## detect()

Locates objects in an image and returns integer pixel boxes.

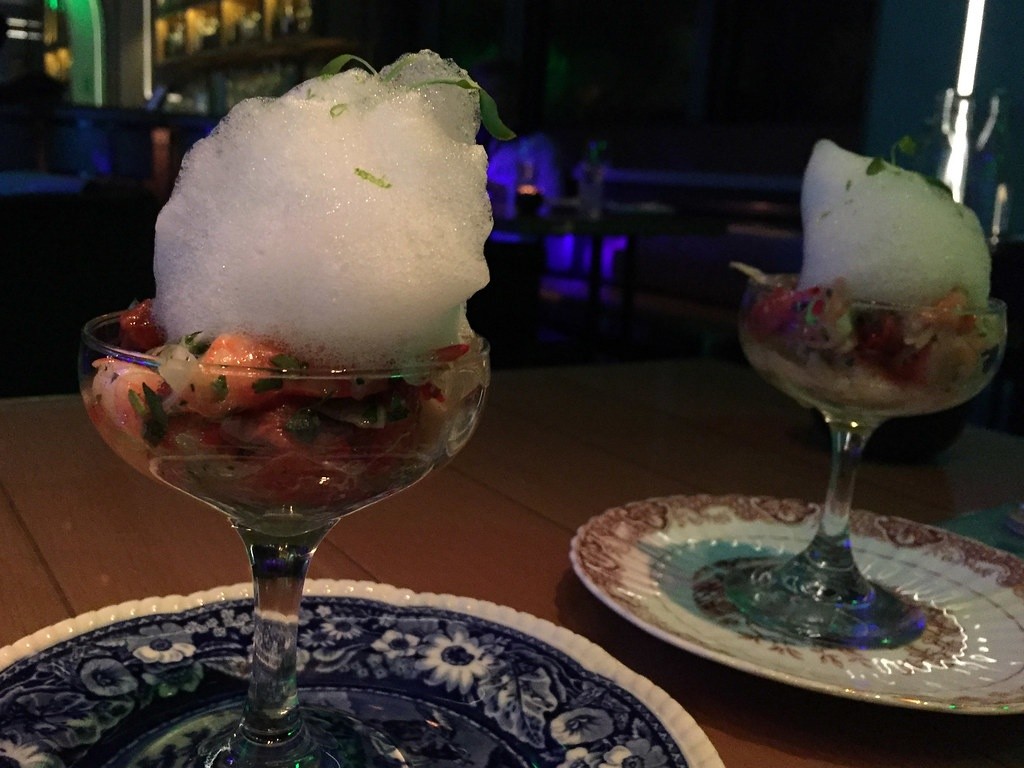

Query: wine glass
[720,283,1009,650]
[75,303,492,768]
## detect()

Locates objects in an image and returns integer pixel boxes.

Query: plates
[1,576,724,768]
[567,493,1024,717]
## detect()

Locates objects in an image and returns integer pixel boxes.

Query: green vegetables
[126,52,515,444]
[865,138,955,203]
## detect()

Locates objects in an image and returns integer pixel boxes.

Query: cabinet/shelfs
[151,1,355,122]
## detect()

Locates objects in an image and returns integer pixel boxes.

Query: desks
[494,207,724,361]
[0,165,136,211]
[1,364,1024,768]
